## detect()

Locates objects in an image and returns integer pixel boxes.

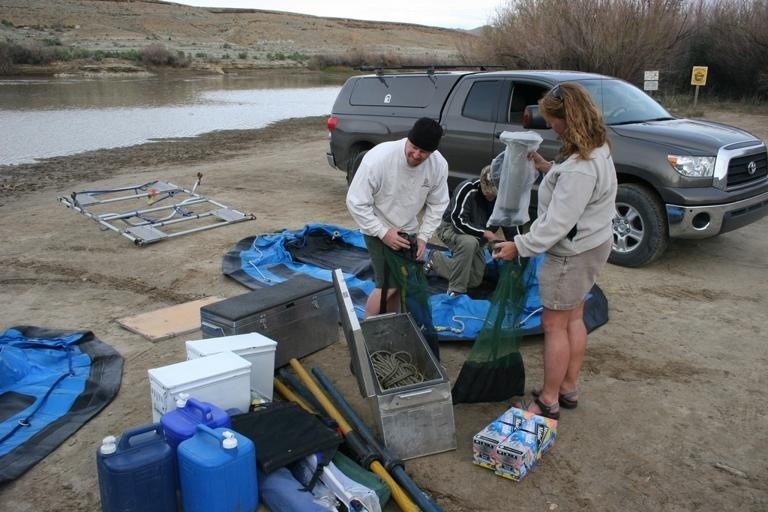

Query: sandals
[532,382,578,410]
[509,397,560,421]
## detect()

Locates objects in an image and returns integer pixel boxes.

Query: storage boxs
[147,350,252,424]
[186,333,278,405]
[332,269,457,461]
[200,273,339,369]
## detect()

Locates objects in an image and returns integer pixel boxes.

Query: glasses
[552,83,564,105]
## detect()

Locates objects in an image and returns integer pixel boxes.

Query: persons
[345,117,452,320]
[487,81,620,422]
[423,164,525,294]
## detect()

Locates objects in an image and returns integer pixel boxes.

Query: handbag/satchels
[231,402,340,471]
[258,448,391,512]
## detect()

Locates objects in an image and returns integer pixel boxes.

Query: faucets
[177,393,191,408]
[220,429,238,449]
[98,434,116,454]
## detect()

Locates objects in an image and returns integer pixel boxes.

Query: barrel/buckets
[176,422,259,512]
[159,395,230,446]
[95,420,175,512]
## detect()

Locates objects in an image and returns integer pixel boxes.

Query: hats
[408,117,443,152]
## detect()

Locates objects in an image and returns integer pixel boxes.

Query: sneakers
[447,287,461,297]
[423,249,439,275]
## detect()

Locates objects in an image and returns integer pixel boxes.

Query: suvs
[325,65,766,268]
